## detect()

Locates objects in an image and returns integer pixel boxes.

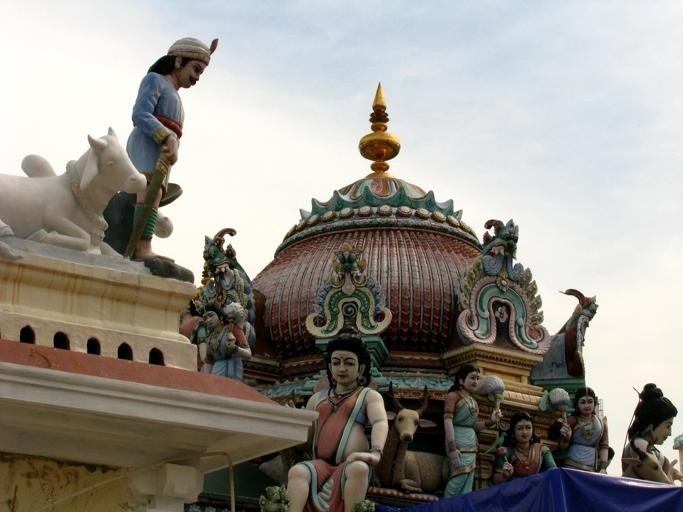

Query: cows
[373,380,438,493]
[1,125,148,260]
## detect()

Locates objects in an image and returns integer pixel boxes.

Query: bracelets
[230,344,239,355]
[369,447,384,458]
[561,434,569,445]
[448,441,456,452]
[197,336,208,345]
[485,420,495,427]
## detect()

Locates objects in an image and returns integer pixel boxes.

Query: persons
[620,383,682,483]
[557,387,609,475]
[490,410,559,483]
[442,363,504,499]
[285,323,390,512]
[194,302,252,383]
[125,35,220,263]
